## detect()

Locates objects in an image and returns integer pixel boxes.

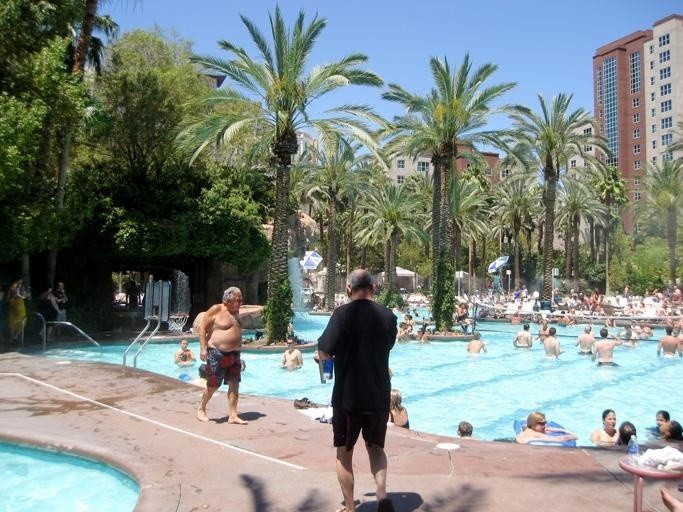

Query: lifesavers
[514,416,576,447]
[645,425,663,441]
[176,358,196,367]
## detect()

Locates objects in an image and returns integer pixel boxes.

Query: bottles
[626,434,641,469]
[323,358,334,380]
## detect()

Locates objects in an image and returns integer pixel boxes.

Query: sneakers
[294,398,319,409]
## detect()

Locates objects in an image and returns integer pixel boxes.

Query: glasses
[537,421,546,425]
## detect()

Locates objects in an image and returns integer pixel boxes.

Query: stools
[618,454,683,512]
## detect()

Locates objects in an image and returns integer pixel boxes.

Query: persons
[172,279,683,449]
[124,281,144,329]
[53,282,69,336]
[658,485,683,512]
[6,274,33,346]
[37,285,63,341]
[312,268,400,511]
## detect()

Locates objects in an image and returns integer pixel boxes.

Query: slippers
[377,498,395,512]
[337,509,357,512]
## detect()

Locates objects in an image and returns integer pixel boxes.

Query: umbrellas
[299,250,324,271]
[488,256,510,274]
